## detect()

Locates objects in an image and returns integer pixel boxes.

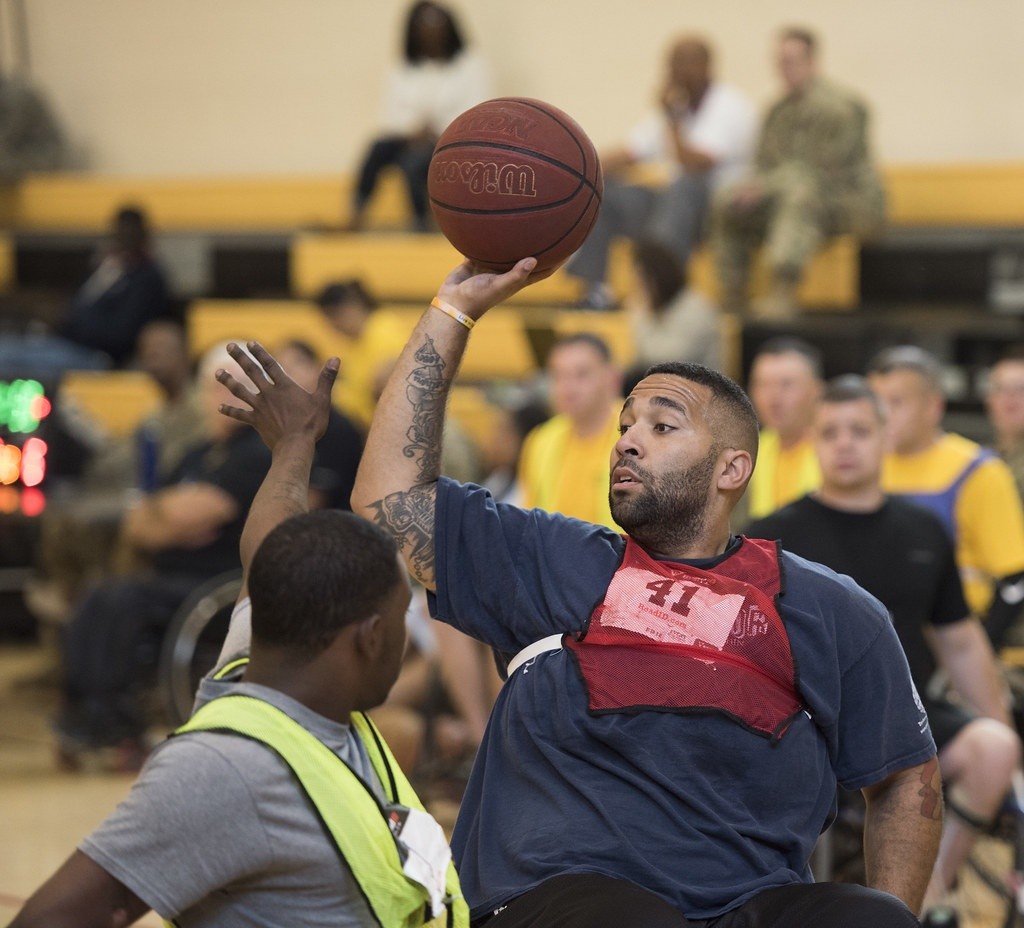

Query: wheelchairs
[29,541,1024,928]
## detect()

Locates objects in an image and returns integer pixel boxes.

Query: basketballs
[426,94,604,277]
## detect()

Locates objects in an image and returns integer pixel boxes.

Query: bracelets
[433,298,475,327]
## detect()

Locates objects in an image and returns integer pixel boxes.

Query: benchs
[0,169,1021,905]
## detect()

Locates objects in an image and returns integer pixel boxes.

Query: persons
[747,374,1018,928]
[7,340,470,928]
[349,1,495,229]
[565,32,884,323]
[351,257,943,928]
[0,208,1024,928]
